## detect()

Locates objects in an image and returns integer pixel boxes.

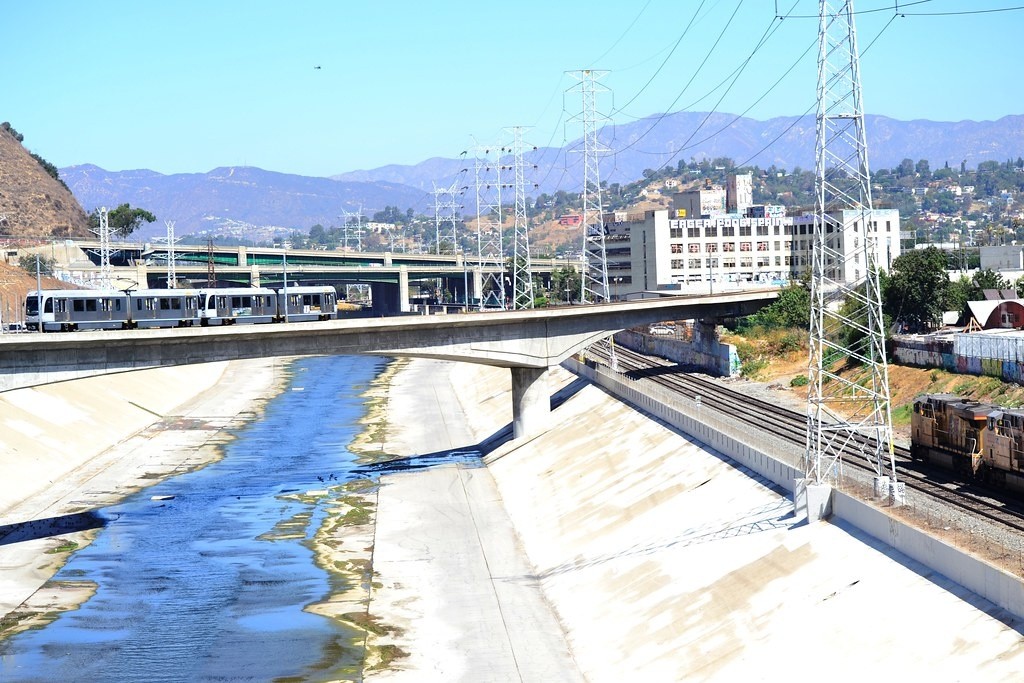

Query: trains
[910,393,1024,499]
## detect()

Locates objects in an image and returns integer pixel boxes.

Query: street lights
[614,275,623,302]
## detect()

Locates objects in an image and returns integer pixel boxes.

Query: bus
[22,285,337,333]
